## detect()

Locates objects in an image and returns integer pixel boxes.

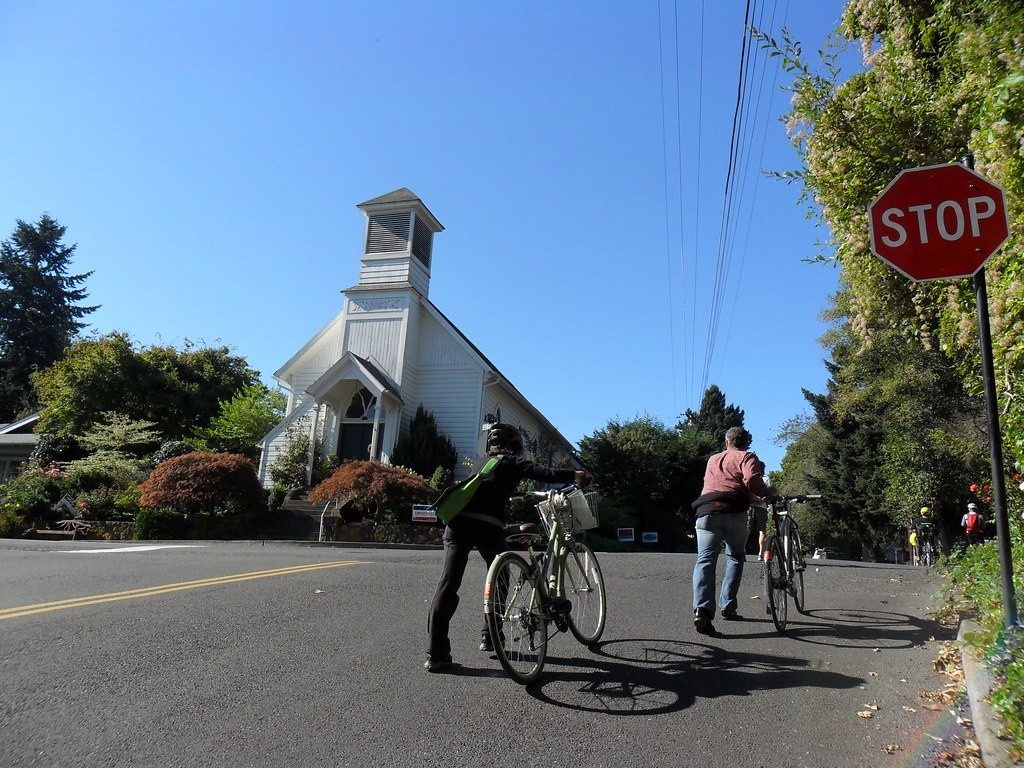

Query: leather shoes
[693,607,714,633]
[721,605,738,619]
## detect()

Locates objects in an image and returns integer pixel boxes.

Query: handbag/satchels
[428,472,484,525]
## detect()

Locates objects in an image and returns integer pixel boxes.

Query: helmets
[485,423,524,454]
[920,506,931,515]
[967,503,977,509]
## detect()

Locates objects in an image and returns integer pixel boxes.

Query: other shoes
[424,649,454,671]
[915,556,920,560]
[480,634,506,652]
[758,554,763,561]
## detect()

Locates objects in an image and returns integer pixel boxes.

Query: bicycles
[482,475,610,686]
[909,518,934,566]
[749,491,828,632]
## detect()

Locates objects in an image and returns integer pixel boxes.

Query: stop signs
[864,160,1011,289]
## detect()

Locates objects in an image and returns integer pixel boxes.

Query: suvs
[811,547,823,560]
[820,546,841,559]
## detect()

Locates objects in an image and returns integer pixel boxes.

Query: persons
[961,503,985,547]
[425,421,584,670]
[743,461,783,561]
[909,507,941,562]
[692,426,779,633]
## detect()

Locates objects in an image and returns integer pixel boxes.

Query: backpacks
[966,512,979,532]
